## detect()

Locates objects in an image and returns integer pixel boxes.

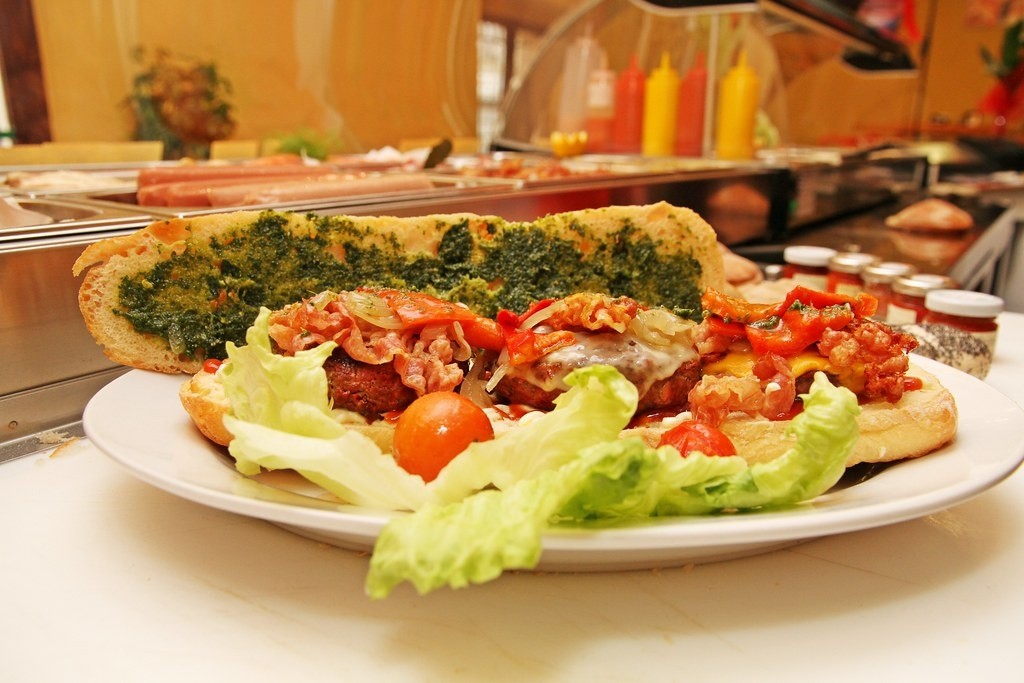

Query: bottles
[560,22,760,158]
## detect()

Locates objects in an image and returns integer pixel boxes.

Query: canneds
[783,244,1004,383]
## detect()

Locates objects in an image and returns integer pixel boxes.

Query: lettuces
[210,304,864,604]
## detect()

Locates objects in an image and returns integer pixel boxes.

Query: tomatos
[391,389,495,484]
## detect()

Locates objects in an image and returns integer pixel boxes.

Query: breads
[181,361,958,472]
[69,200,724,376]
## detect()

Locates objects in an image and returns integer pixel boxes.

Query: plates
[81,351,1024,572]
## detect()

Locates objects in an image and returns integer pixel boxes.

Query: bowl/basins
[0,155,724,241]
